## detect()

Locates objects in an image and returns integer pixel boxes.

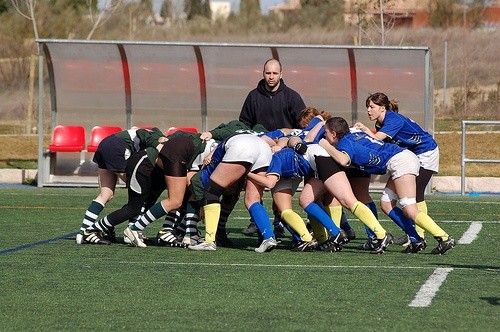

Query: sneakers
[392,236,410,245]
[123,227,146,248]
[357,237,373,250]
[181,234,204,245]
[432,236,456,255]
[172,230,185,242]
[288,238,319,251]
[273,224,285,237]
[107,229,117,243]
[313,238,342,252]
[215,229,232,247]
[188,240,216,251]
[241,222,258,236]
[254,237,278,253]
[156,229,182,246]
[371,232,394,254]
[83,229,111,245]
[346,228,356,241]
[404,239,426,253]
[328,230,350,248]
[75,234,84,245]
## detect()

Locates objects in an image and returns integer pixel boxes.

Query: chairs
[58,57,427,109]
[48,126,197,174]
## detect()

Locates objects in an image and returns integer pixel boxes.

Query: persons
[320,117,456,254]
[240,59,306,237]
[77,107,407,252]
[355,92,439,246]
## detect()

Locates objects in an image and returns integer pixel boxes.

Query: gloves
[295,143,308,155]
